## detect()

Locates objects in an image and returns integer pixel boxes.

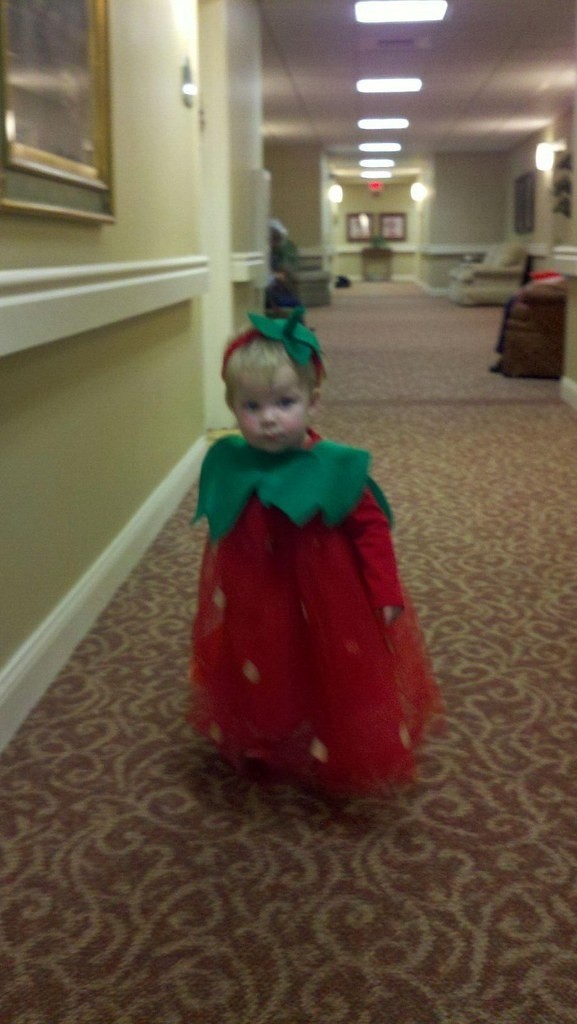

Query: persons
[189,305,443,796]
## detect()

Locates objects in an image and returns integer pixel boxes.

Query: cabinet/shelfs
[505,289,564,382]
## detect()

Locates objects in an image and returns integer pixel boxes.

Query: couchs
[449,244,528,306]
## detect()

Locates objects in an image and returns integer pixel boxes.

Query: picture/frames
[378,212,407,242]
[0,0,118,226]
[346,212,374,242]
